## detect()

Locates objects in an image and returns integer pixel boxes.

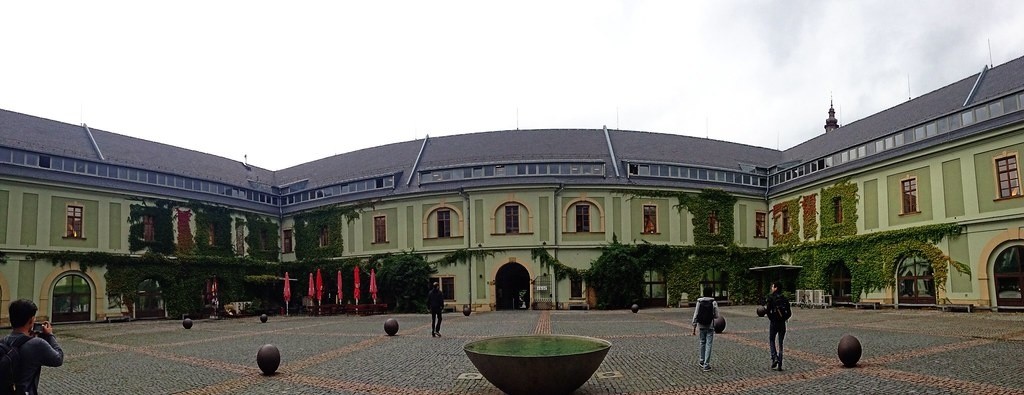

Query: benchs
[854,302,879,310]
[679,301,697,307]
[717,301,731,306]
[108,316,130,323]
[937,304,973,313]
[568,304,589,310]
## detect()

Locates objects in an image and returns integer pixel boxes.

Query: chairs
[306,304,388,316]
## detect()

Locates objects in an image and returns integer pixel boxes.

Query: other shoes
[778,365,783,371]
[432,332,435,337]
[436,332,441,336]
[771,357,780,368]
[703,364,712,372]
[699,362,704,367]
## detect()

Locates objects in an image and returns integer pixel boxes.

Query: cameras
[31,322,47,333]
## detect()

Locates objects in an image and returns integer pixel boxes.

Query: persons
[766,282,791,371]
[692,286,720,371]
[0,298,63,395]
[66,295,71,312]
[426,282,444,338]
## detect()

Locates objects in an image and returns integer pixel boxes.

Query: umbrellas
[308,273,316,313]
[369,269,378,305]
[338,270,343,306]
[283,272,291,315]
[353,266,360,314]
[315,269,323,315]
[211,278,218,316]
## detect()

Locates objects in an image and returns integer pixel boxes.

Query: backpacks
[769,294,791,322]
[697,300,715,325]
[0,335,38,395]
